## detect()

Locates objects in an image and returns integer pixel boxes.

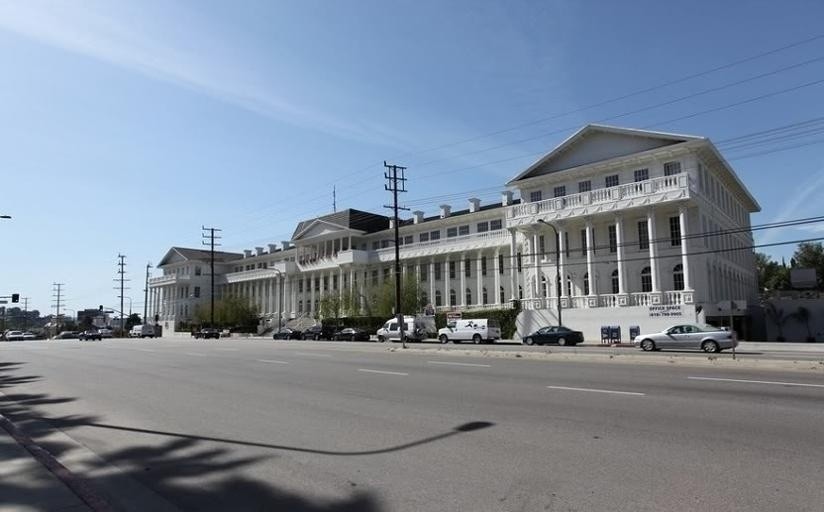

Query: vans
[376,315,429,343]
[438,318,502,347]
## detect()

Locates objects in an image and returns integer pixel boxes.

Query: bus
[321,315,391,341]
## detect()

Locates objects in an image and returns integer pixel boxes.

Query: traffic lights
[99,305,103,312]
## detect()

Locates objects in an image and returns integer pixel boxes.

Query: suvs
[128,325,155,339]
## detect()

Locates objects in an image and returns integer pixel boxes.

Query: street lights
[117,295,132,320]
[63,308,75,320]
[262,267,281,333]
[536,218,562,326]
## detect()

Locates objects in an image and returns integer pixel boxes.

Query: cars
[191,328,220,339]
[521,325,583,348]
[0,327,115,341]
[633,321,738,354]
[301,324,324,340]
[273,327,301,340]
[333,327,370,341]
